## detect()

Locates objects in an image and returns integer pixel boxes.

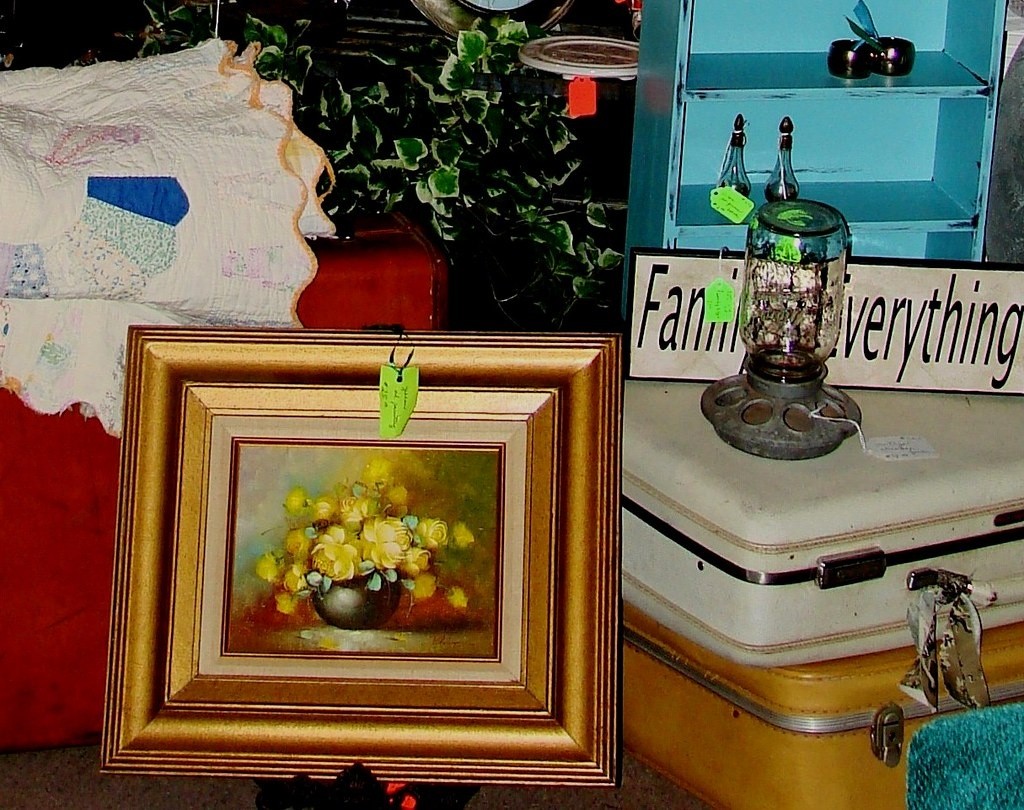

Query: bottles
[716,114,751,200]
[763,117,800,203]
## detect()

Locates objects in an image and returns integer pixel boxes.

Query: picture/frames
[101,320,624,789]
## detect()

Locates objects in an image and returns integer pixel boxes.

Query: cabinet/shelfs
[622,0,1007,326]
